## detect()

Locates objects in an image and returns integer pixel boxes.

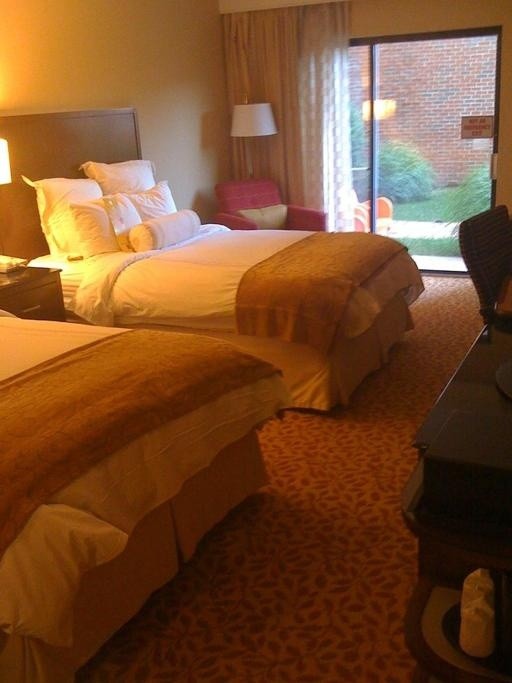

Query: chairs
[458,204,511,331]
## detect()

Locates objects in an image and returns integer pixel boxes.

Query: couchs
[352,188,393,232]
[213,178,327,229]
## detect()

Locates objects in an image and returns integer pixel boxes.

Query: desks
[397,318,510,682]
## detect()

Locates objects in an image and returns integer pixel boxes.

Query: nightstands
[1,266,67,322]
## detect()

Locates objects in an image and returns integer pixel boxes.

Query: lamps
[230,102,279,177]
[0,136,14,184]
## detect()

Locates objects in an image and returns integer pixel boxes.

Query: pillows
[129,207,202,254]
[91,191,145,252]
[77,158,156,195]
[66,196,120,258]
[235,202,288,230]
[128,179,178,221]
[21,175,102,259]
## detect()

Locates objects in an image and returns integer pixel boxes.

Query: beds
[0,309,285,683]
[27,223,425,413]
